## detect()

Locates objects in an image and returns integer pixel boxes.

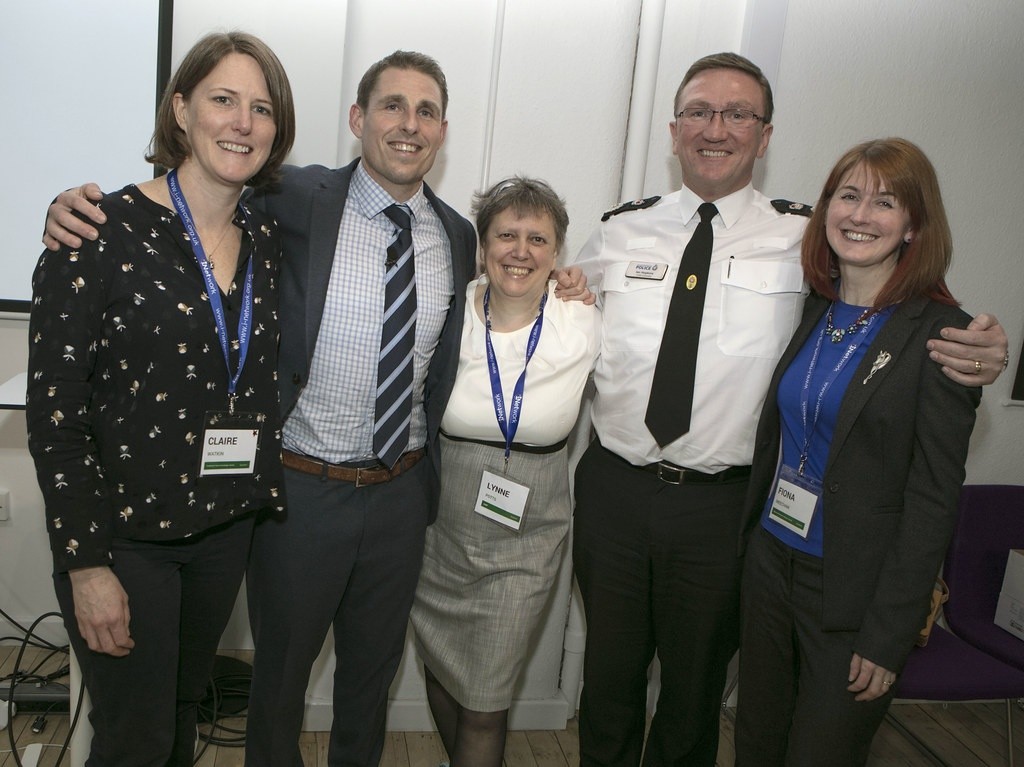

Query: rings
[975,361,981,375]
[884,682,892,685]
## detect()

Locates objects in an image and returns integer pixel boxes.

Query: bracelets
[1003,351,1008,371]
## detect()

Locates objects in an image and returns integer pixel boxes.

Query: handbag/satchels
[916,576,949,646]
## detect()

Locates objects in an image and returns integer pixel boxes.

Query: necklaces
[196,216,233,270]
[826,301,881,343]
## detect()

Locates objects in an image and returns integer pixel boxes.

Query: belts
[603,448,751,486]
[282,448,426,488]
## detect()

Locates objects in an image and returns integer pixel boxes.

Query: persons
[407,175,603,767]
[25,31,285,767]
[569,53,1007,767]
[41,51,594,767]
[734,135,983,767]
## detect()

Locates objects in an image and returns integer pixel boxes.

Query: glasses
[675,108,766,128]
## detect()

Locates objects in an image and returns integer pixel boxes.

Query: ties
[372,204,417,470]
[644,202,720,448]
